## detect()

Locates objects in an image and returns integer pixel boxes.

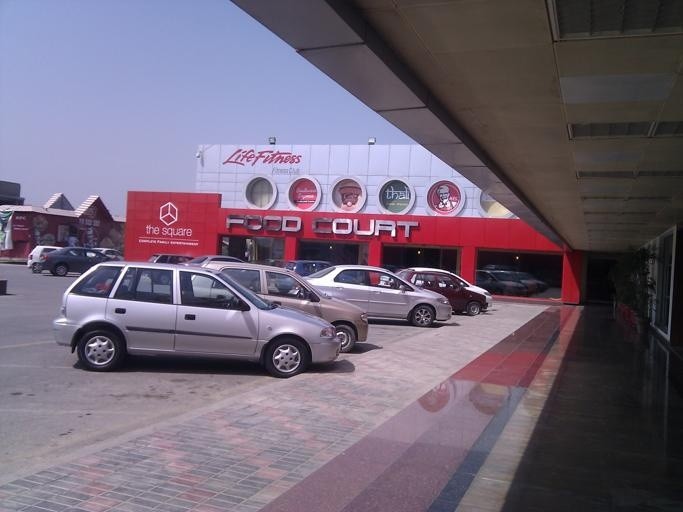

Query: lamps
[368,136,376,144]
[268,136,277,144]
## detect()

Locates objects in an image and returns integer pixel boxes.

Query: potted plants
[615,246,658,335]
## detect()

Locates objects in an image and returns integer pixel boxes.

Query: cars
[27,246,124,277]
[475,267,549,296]
